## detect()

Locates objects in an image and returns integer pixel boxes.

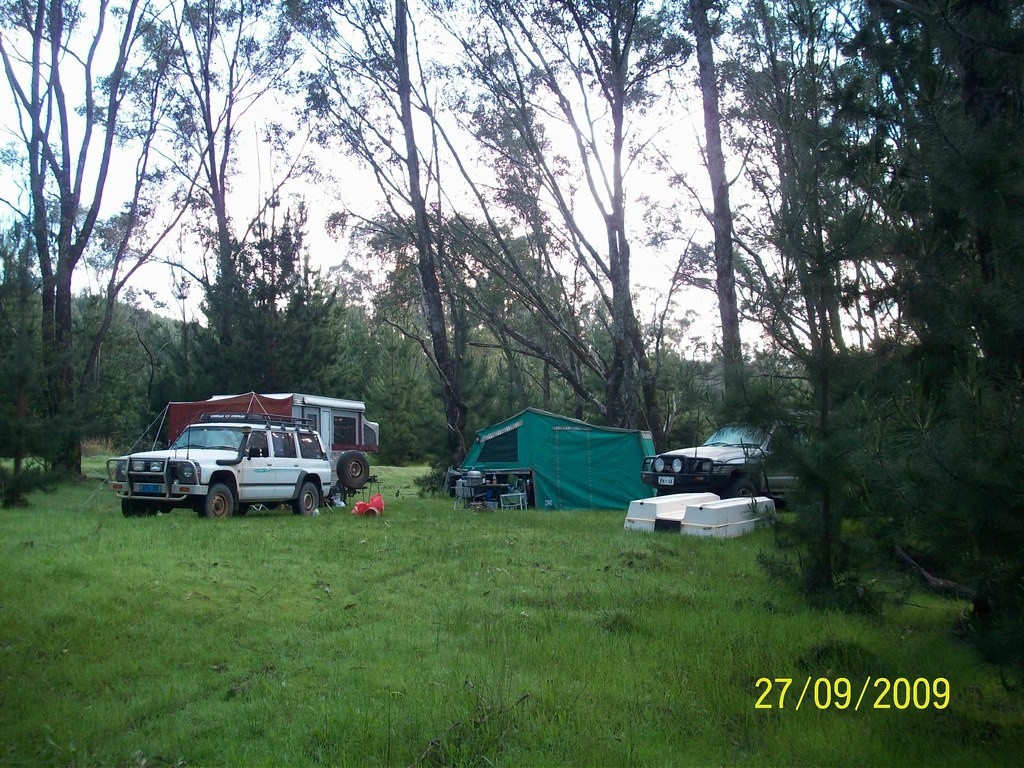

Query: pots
[467,471,482,485]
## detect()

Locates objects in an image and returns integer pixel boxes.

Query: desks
[451,483,529,510]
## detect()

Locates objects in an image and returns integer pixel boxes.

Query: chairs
[454,479,486,510]
[501,478,527,512]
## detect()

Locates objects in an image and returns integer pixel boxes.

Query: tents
[458,407,658,510]
[166,391,294,447]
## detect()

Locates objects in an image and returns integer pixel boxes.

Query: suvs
[640,410,839,509]
[106,411,331,520]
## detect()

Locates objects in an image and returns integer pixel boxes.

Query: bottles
[482,475,486,485]
[493,474,497,485]
